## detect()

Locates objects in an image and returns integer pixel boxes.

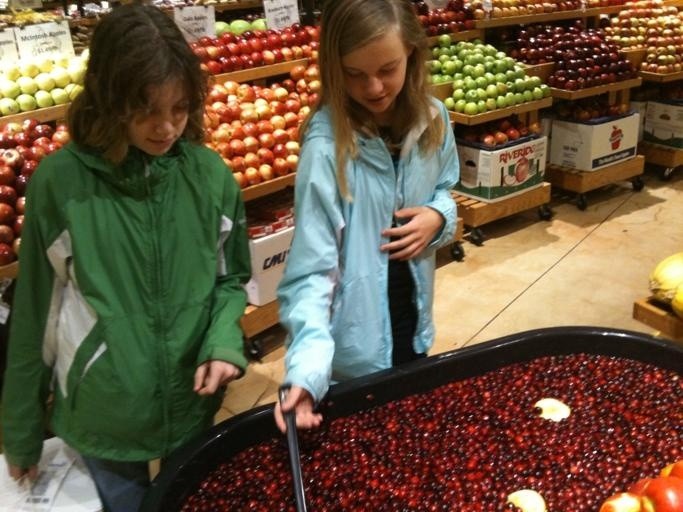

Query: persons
[0,3,254,512]
[270,0,463,434]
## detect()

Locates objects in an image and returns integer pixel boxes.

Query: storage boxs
[454,99,683,206]
[238,225,299,309]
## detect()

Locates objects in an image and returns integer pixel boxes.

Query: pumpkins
[663,280,683,319]
[649,252,683,305]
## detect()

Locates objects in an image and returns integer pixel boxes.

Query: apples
[516,157,529,182]
[188,20,320,189]
[0,48,90,267]
[415,0,683,148]
